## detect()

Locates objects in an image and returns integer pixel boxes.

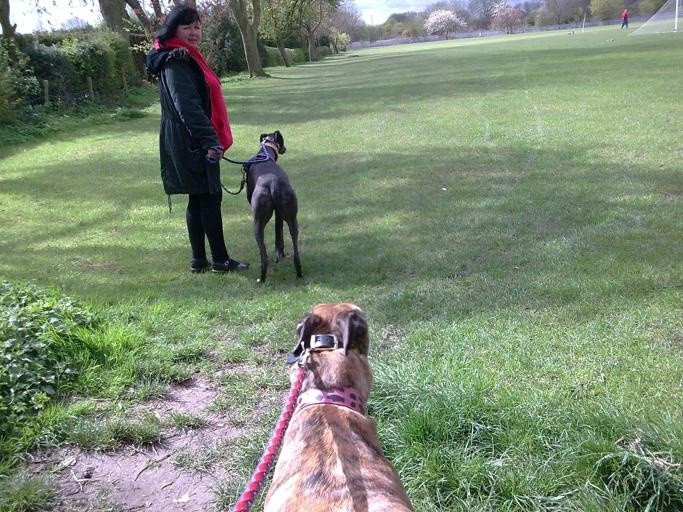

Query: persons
[144,5,248,276]
[621,9,628,30]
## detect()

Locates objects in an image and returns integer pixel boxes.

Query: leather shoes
[190,258,211,272]
[211,258,248,273]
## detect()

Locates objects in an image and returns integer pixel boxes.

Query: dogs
[242,130,305,283]
[264,299,413,512]
